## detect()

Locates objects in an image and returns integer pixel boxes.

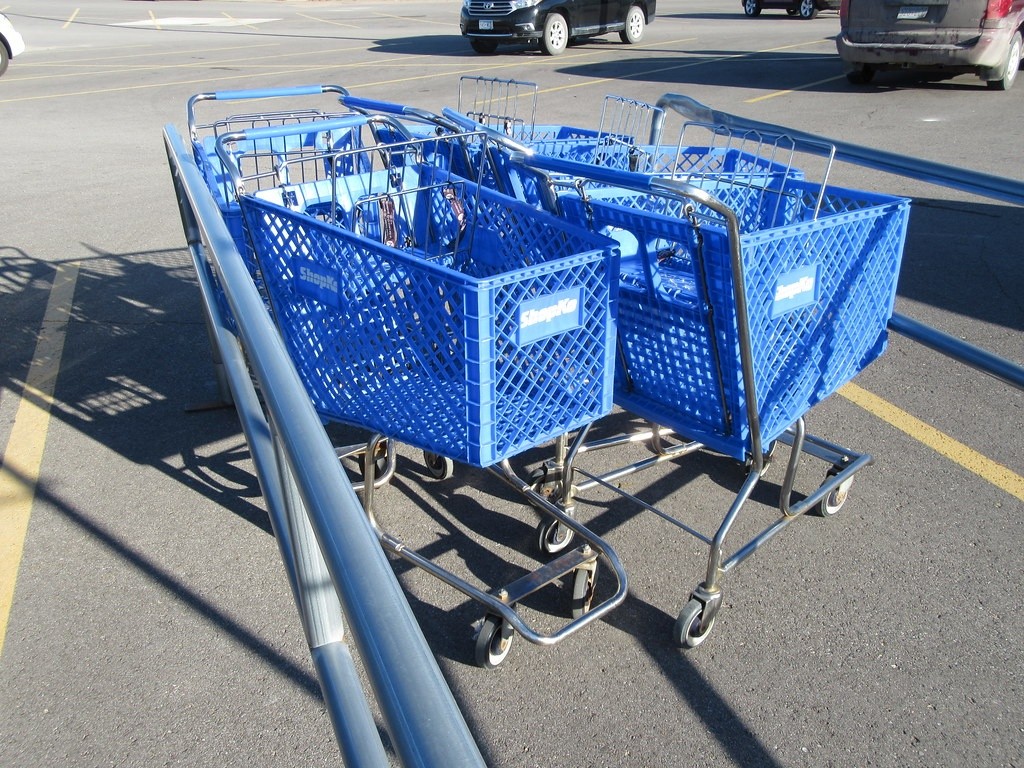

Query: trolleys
[182,75,914,673]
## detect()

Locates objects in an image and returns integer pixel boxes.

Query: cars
[834,0,1024,93]
[456,0,657,58]
[739,0,841,20]
